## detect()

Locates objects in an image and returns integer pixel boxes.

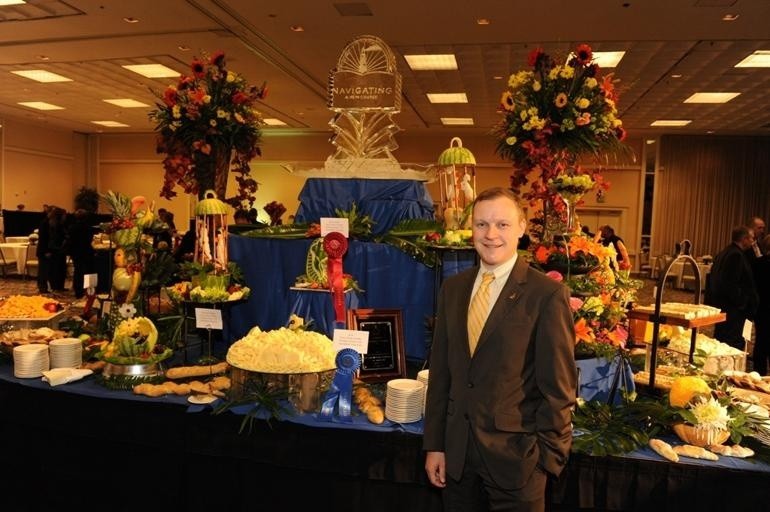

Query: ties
[464,271,496,359]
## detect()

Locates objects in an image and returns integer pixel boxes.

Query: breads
[351,378,385,424]
[166,362,229,379]
[650,438,679,463]
[711,445,756,459]
[674,444,719,463]
[133,376,231,397]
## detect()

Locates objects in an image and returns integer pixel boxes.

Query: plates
[739,403,769,419]
[187,395,218,404]
[12,337,83,379]
[384,369,430,424]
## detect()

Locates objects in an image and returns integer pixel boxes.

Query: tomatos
[43,303,58,314]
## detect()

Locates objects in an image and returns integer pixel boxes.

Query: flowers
[148,48,272,210]
[492,39,636,360]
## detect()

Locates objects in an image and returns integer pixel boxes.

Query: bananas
[136,206,155,228]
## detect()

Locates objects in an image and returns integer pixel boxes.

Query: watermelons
[193,199,227,216]
[190,273,231,291]
[118,316,159,357]
[437,136,478,167]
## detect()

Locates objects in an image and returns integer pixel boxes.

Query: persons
[233,209,249,223]
[749,233,770,375]
[249,208,262,224]
[160,212,176,249]
[600,225,630,265]
[419,187,577,509]
[36,202,71,292]
[705,225,759,348]
[153,208,166,249]
[581,226,596,237]
[745,216,768,270]
[68,208,105,299]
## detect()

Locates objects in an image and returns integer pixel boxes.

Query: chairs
[0,217,74,280]
[641,238,714,292]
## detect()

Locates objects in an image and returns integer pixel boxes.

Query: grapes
[263,200,288,220]
[548,173,595,206]
[109,219,134,233]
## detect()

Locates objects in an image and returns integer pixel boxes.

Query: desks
[1,363,770,511]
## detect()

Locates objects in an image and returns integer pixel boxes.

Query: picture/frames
[344,307,408,387]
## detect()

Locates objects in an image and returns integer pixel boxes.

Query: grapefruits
[112,268,133,291]
[669,375,712,408]
